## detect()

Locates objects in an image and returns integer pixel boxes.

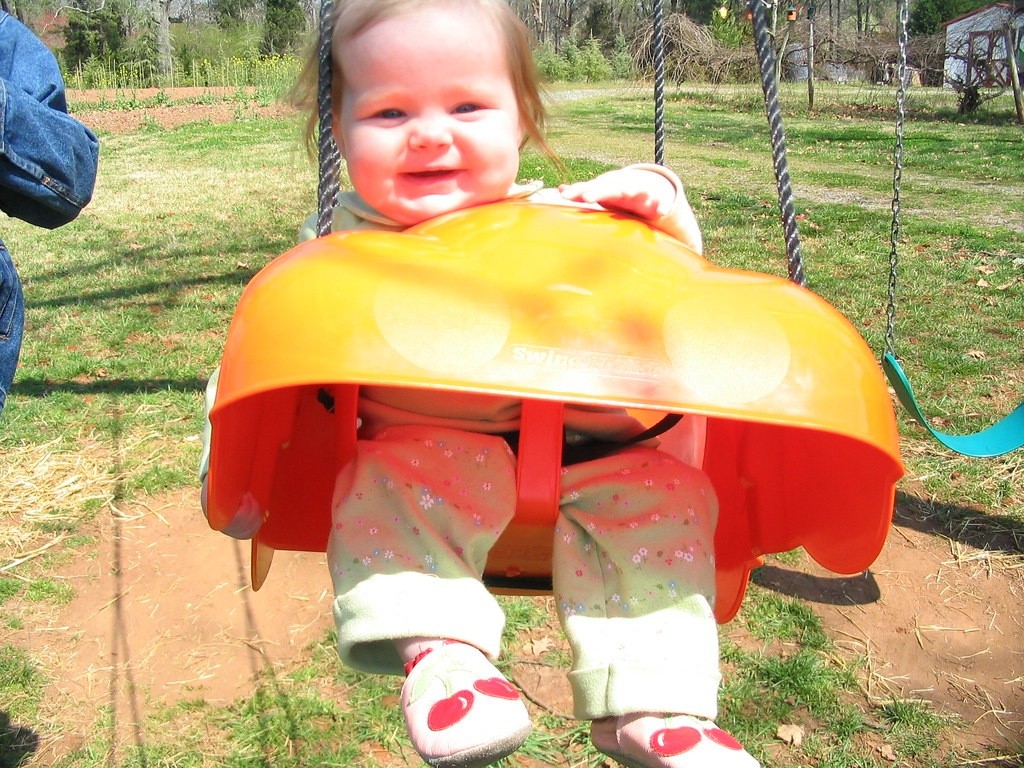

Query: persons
[1,0,102,417]
[198,2,762,768]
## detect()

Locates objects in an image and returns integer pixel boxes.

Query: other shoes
[591,709,759,768]
[400,643,531,768]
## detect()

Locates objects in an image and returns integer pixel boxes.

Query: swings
[877,1,1024,458]
[204,0,907,624]
